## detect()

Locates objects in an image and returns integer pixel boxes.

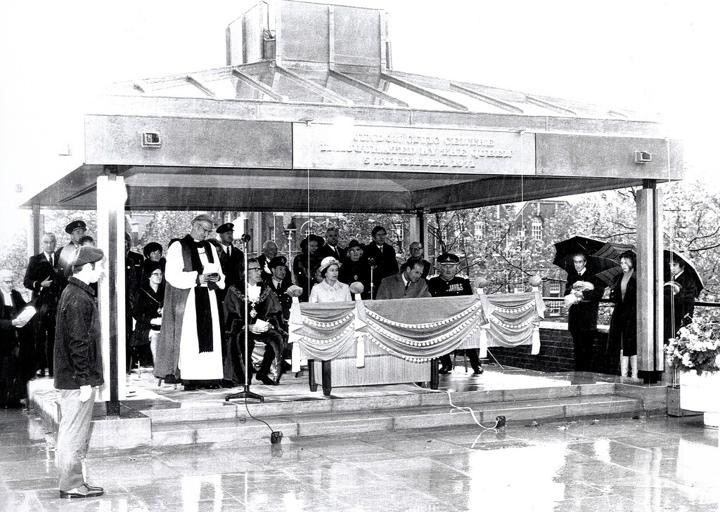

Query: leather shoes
[439,362,452,375]
[471,362,484,375]
[248,370,277,386]
[61,483,104,499]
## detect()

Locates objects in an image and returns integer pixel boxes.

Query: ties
[334,248,339,256]
[49,255,53,268]
[405,281,410,291]
[380,247,382,252]
[578,272,582,278]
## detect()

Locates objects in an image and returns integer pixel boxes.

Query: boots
[627,354,639,379]
[617,348,629,378]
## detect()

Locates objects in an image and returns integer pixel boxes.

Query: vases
[679,368,720,412]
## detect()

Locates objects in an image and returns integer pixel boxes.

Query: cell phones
[208,273,218,276]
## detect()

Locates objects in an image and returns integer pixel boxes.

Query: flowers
[664,309,720,375]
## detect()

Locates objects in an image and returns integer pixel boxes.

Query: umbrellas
[553,233,606,290]
[667,247,710,298]
[591,239,638,287]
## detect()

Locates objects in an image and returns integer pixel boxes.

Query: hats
[344,240,366,250]
[267,256,287,269]
[65,220,87,235]
[68,246,105,266]
[318,256,342,272]
[217,223,234,233]
[299,233,325,249]
[437,253,460,265]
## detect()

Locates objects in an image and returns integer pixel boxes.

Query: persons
[564,251,604,372]
[120,215,483,381]
[0,218,94,408]
[610,252,638,379]
[53,246,104,500]
[658,259,700,343]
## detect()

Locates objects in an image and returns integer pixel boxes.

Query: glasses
[247,267,263,273]
[198,224,213,236]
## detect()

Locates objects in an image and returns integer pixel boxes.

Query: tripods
[225,242,265,402]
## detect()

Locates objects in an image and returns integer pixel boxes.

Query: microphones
[369,259,376,266]
[241,234,251,242]
